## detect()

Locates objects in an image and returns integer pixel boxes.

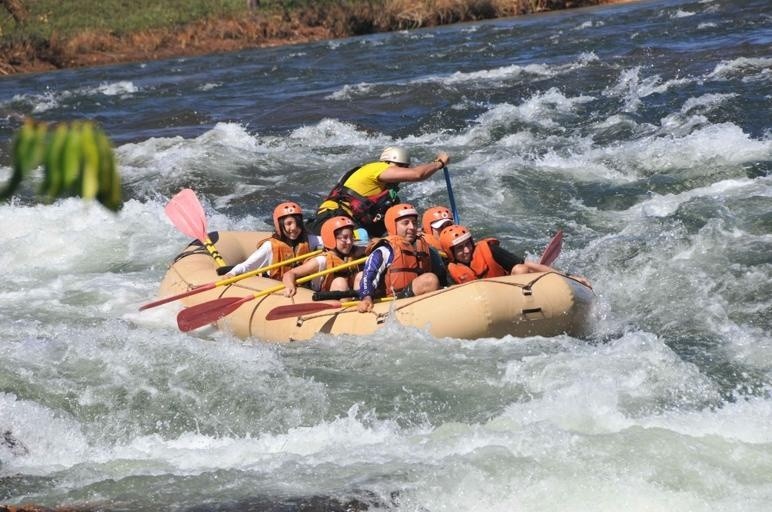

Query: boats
[158,232,597,351]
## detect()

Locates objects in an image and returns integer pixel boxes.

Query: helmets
[273,202,474,262]
[379,146,412,165]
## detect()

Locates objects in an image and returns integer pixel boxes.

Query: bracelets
[433,159,445,169]
[566,271,572,279]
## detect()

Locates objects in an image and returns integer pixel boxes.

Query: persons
[220,201,324,290]
[315,145,450,234]
[358,204,450,313]
[413,206,454,256]
[281,217,367,303]
[436,225,591,290]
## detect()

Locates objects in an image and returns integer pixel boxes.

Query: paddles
[266,297,398,321]
[139,248,325,310]
[177,257,369,332]
[539,230,562,264]
[165,188,228,266]
